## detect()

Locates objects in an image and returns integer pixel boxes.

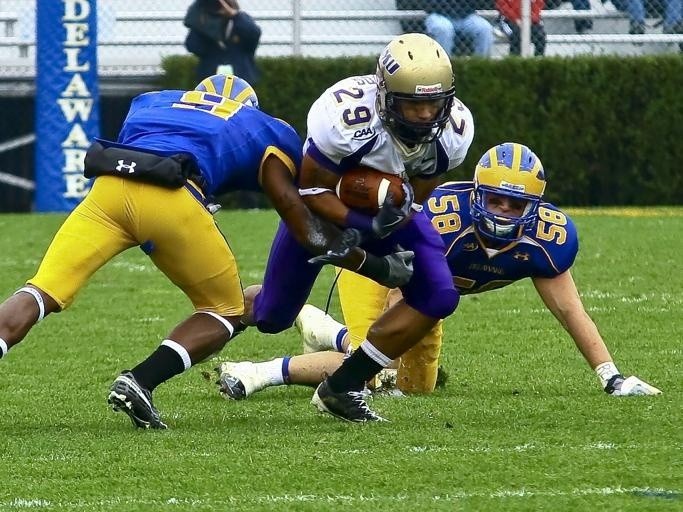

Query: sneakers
[309,376,390,423]
[213,360,258,400]
[294,302,328,355]
[105,368,168,428]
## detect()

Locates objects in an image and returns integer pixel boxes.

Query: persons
[183,0,261,88]
[0,72,417,432]
[195,33,475,423]
[393,0,683,63]
[215,142,664,403]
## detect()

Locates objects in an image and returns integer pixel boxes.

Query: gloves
[373,180,415,241]
[304,226,361,265]
[377,369,405,396]
[592,362,662,397]
[355,243,415,290]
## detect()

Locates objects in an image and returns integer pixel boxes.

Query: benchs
[0,0,681,59]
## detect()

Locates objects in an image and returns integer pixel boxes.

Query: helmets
[375,32,455,123]
[192,73,259,113]
[473,141,546,227]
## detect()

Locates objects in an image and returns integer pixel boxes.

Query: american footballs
[336,170,410,215]
